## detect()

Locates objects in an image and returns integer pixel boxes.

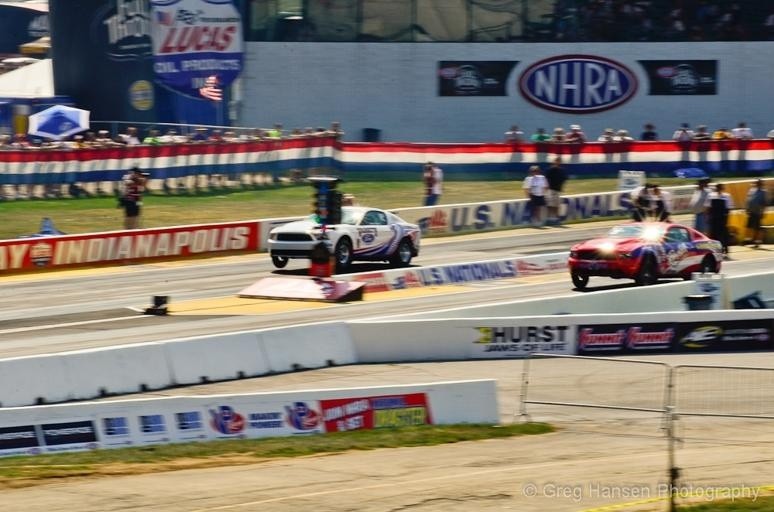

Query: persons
[1,121,344,199]
[506,120,773,260]
[120,167,145,229]
[341,194,357,205]
[424,162,443,206]
[423,165,437,196]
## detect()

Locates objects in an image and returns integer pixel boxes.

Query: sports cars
[567,219,723,290]
[267,205,422,270]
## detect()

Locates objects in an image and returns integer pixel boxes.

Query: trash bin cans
[362,128,381,142]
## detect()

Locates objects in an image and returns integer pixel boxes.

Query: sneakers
[529,218,564,230]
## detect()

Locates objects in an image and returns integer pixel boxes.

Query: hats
[537,122,728,138]
[0,125,239,149]
